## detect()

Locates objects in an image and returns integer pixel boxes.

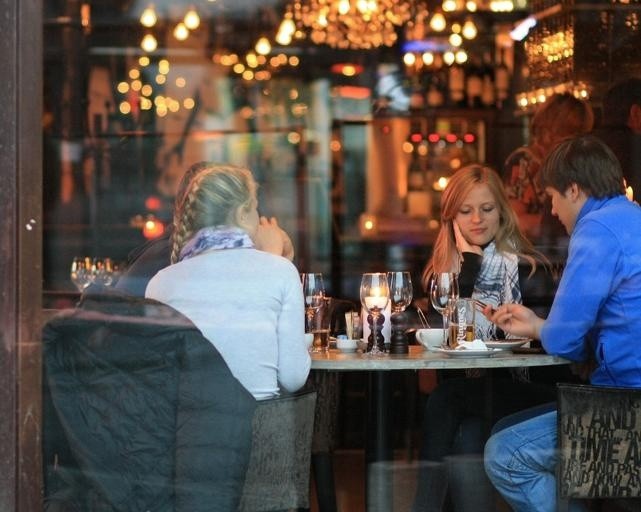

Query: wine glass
[360,272,389,358]
[431,272,459,353]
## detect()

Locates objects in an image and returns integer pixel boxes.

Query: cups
[448,299,476,348]
[386,272,413,312]
[305,296,332,353]
[416,329,450,351]
[302,272,324,353]
[70,254,115,292]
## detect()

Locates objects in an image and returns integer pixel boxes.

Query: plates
[443,348,503,357]
[481,339,533,351]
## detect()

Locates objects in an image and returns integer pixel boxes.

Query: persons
[483,135,641,512]
[416,162,556,511]
[141,163,343,511]
[601,79,641,206]
[531,91,596,284]
[110,161,213,299]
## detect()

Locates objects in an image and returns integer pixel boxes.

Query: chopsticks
[475,298,510,322]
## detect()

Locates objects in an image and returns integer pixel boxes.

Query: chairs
[556,383,641,512]
[42,308,339,512]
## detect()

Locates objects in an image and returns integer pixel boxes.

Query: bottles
[367,306,409,354]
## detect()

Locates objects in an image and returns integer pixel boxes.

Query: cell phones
[476,300,496,313]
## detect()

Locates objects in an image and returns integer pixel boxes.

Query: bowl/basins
[336,339,361,353]
